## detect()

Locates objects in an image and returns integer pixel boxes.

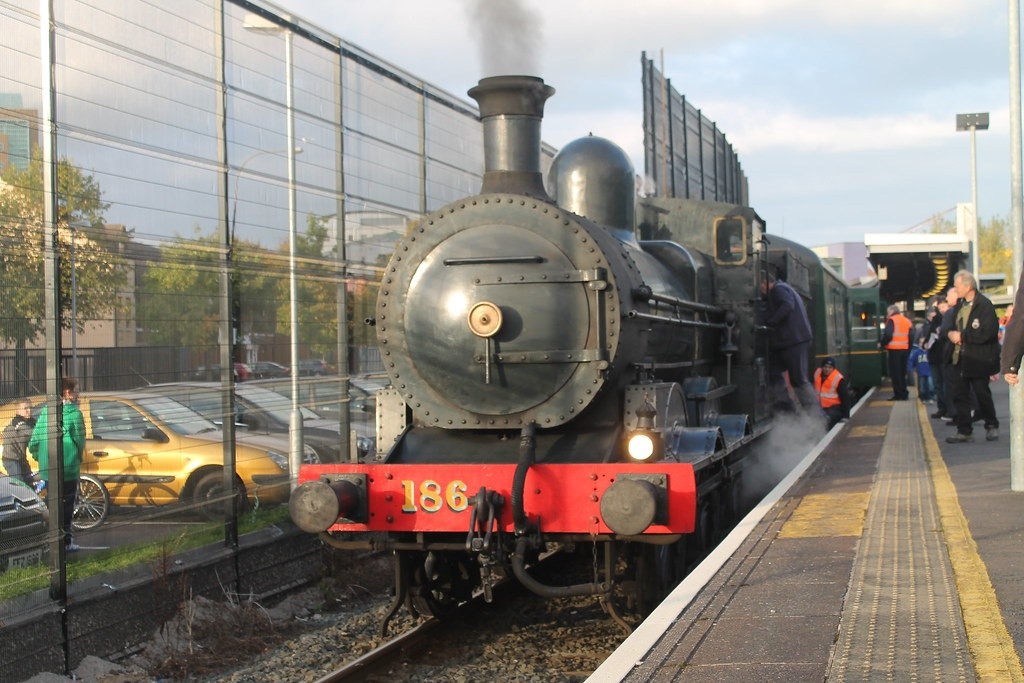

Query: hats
[821,357,836,368]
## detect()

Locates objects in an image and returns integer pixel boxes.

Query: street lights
[243,9,305,493]
[229,146,305,269]
[955,112,990,291]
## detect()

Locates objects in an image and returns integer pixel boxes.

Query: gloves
[840,418,849,423]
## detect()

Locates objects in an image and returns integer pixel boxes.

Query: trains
[287,75,884,635]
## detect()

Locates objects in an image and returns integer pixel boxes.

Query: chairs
[103,405,123,432]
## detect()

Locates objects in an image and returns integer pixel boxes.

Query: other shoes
[946,434,975,443]
[986,425,999,441]
[972,410,984,421]
[931,411,956,426]
[888,391,909,400]
[923,399,934,404]
[65,544,80,551]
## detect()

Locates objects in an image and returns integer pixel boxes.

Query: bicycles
[32,469,112,532]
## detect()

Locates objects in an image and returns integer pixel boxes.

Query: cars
[0,470,51,575]
[298,359,329,377]
[186,364,254,381]
[1,392,321,520]
[250,362,290,380]
[94,372,391,460]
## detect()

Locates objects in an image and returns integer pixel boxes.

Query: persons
[906,264,1024,443]
[812,357,850,428]
[4,378,86,551]
[877,304,914,401]
[757,269,831,424]
